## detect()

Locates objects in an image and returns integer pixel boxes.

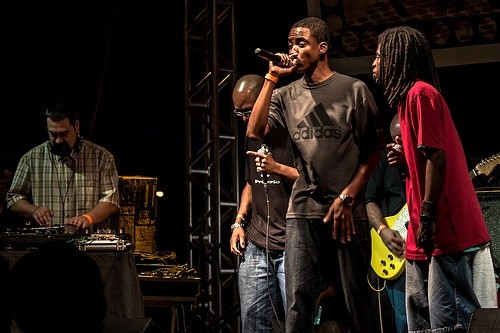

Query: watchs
[338,194,355,208]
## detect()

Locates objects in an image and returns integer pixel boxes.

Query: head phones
[47,135,84,151]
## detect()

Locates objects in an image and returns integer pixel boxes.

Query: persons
[246,16,380,333]
[371,27,493,333]
[365,113,411,333]
[228,76,291,333]
[6,101,120,229]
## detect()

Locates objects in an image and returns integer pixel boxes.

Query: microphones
[255,47,292,68]
[258,148,269,188]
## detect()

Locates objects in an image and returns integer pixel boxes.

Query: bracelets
[82,214,93,224]
[420,200,438,223]
[237,214,247,220]
[377,224,386,236]
[231,223,244,230]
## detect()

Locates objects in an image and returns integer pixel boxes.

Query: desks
[0,236,144,333]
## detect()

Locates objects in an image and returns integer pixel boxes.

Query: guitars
[370,153,500,281]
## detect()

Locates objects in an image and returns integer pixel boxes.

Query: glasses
[234,110,252,117]
